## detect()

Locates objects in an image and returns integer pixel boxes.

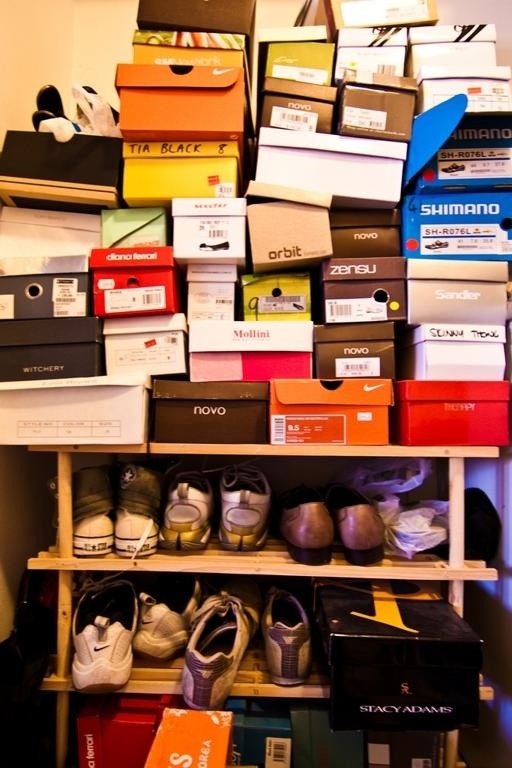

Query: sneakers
[72,570,313,710]
[113,466,156,558]
[219,465,268,548]
[47,466,112,556]
[158,465,213,550]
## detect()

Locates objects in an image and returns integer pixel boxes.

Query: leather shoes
[32,84,63,131]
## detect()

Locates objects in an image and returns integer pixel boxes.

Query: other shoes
[268,470,335,565]
[314,469,385,564]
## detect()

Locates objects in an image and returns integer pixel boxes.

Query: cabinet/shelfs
[28,443,500,768]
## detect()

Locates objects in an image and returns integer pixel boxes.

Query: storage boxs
[307,582,488,730]
[369,728,445,768]
[75,693,293,768]
[292,707,368,768]
[0,0,512,445]
